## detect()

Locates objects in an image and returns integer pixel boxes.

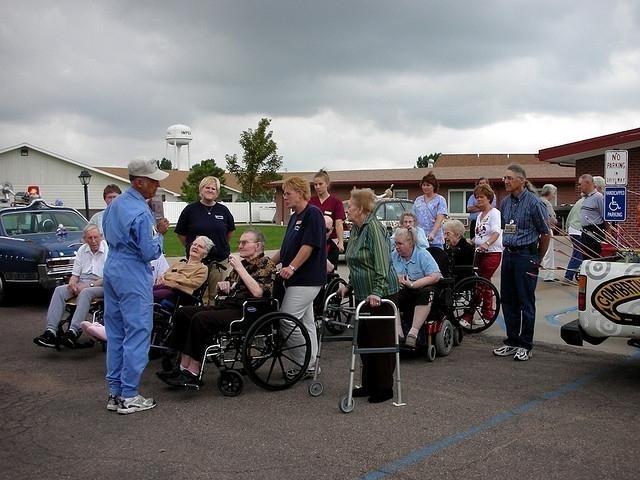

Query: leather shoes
[368,390,393,403]
[352,386,371,397]
[474,316,491,325]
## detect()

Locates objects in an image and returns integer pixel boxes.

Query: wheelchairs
[442,242,501,335]
[397,248,465,362]
[55,259,312,397]
[310,272,354,334]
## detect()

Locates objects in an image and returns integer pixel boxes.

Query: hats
[128,157,170,180]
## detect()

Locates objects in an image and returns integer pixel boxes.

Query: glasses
[237,240,257,246]
[502,176,513,182]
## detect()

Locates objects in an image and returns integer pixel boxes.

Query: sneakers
[156,367,181,380]
[405,334,418,347]
[107,393,117,411]
[287,366,321,380]
[493,342,519,356]
[166,370,199,386]
[514,347,533,361]
[458,318,470,328]
[116,394,157,415]
[33,321,107,348]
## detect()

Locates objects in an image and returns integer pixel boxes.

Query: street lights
[78,170,91,221]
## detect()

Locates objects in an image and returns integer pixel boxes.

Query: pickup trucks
[558,252,640,348]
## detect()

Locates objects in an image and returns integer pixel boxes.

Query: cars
[0,197,96,296]
[337,198,421,251]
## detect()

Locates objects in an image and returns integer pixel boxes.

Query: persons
[81,235,215,343]
[33,223,109,350]
[89,184,122,228]
[99,158,171,416]
[157,164,605,387]
